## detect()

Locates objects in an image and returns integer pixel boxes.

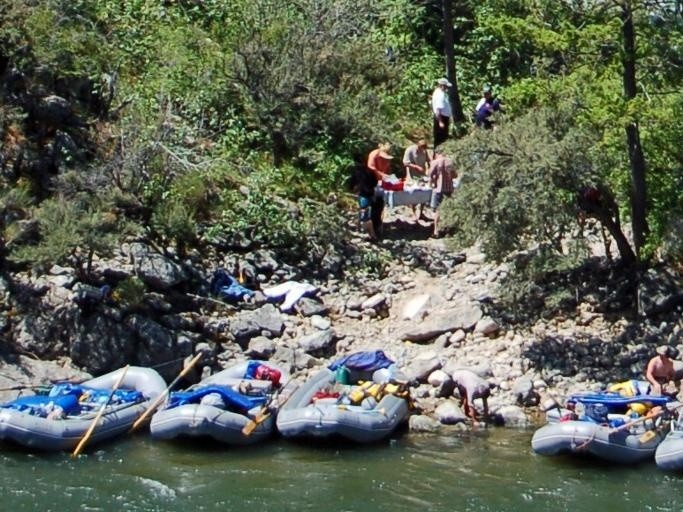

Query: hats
[378,142,394,160]
[438,78,453,87]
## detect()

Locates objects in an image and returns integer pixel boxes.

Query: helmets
[631,402,646,415]
[655,344,669,355]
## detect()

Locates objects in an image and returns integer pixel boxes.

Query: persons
[402,138,436,223]
[430,77,452,149]
[427,150,460,240]
[347,164,378,242]
[642,345,678,400]
[362,141,399,244]
[450,368,491,425]
[475,97,495,128]
[475,87,499,112]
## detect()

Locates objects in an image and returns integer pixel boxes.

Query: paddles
[242,372,299,435]
[127,352,202,432]
[74,363,130,457]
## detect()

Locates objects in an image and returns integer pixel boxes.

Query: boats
[1,366,170,452]
[150,360,299,445]
[276,349,410,442]
[532,379,683,470]
